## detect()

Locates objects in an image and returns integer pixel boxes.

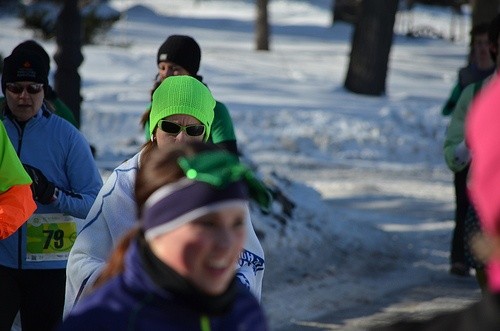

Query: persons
[58,140,270,331]
[443,6,499,307]
[1,120,38,240]
[0,39,79,129]
[63,75,265,321]
[138,34,240,162]
[0,53,104,331]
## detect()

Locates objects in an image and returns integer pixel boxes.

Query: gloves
[21,164,58,207]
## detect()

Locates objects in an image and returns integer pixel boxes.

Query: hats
[150,75,216,144]
[158,35,201,75]
[2,54,49,83]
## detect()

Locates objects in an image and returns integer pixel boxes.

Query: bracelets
[50,188,57,202]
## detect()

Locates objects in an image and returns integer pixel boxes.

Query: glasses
[158,120,206,136]
[5,83,44,94]
